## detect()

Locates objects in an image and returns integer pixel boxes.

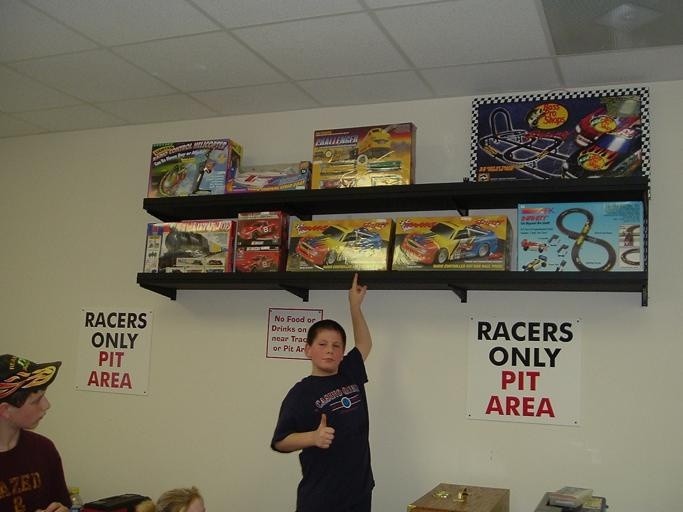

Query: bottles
[69,487,83,512]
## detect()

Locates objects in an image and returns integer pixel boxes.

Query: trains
[348,127,392,160]
[159,231,227,268]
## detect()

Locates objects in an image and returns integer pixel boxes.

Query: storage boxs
[143,220,237,272]
[516,201,643,273]
[391,215,512,271]
[283,219,396,271]
[232,211,288,272]
[146,122,418,198]
[470,86,651,200]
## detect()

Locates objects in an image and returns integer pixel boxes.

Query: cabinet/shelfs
[136,178,648,306]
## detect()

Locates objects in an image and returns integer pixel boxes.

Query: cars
[295,224,383,265]
[563,115,643,179]
[238,220,276,240]
[401,220,499,265]
[236,254,275,272]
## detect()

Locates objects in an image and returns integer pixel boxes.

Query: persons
[0,353,71,511]
[135,485,205,512]
[270,273,374,511]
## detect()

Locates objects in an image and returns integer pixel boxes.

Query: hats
[0,354,63,400]
